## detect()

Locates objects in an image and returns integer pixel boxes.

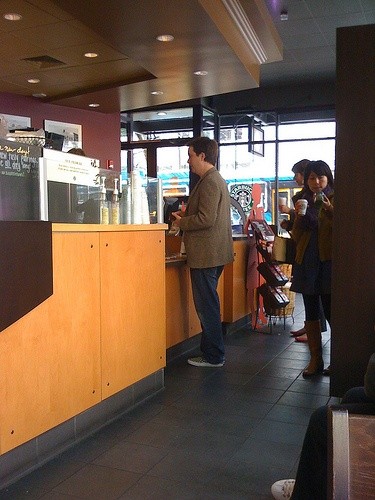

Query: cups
[279,197,286,206]
[131,169,151,225]
[296,199,308,216]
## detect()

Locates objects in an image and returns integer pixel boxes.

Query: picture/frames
[0,113,31,132]
[43,118,83,152]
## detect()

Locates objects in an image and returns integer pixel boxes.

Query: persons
[293,160,335,378]
[270,350,375,500]
[280,159,327,342]
[171,137,234,367]
[68,148,85,156]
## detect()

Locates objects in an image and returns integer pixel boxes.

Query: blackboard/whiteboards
[0,137,41,221]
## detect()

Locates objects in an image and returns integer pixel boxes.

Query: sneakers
[187,355,225,366]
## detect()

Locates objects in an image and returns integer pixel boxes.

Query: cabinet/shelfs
[247,219,291,334]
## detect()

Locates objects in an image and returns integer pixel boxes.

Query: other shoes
[303,360,324,377]
[290,330,303,336]
[296,336,308,342]
[323,366,331,374]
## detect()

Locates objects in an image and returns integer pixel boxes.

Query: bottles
[100,177,110,224]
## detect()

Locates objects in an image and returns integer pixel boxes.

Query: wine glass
[110,178,122,225]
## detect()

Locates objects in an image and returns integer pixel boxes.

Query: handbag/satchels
[273,226,295,263]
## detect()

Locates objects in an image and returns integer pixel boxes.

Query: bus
[118,170,302,236]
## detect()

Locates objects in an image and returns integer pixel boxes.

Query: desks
[327,403,375,500]
